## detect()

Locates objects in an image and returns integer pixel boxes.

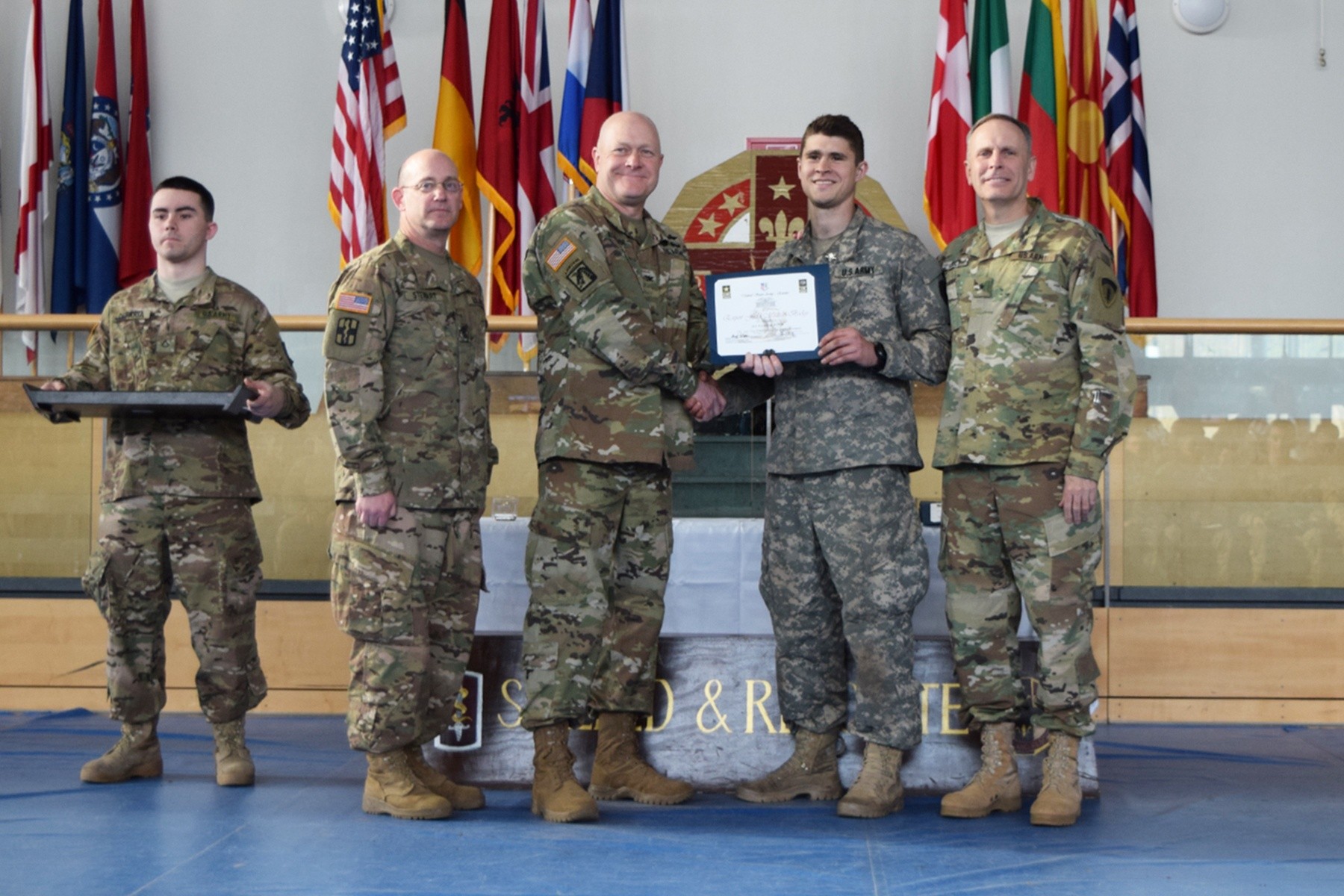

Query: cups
[1132,375,1152,418]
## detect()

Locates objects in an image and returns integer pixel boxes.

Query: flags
[920,2,1157,370]
[15,0,155,379]
[325,2,624,346]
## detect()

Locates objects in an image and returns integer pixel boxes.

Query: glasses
[400,179,464,194]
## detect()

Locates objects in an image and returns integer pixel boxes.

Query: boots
[78,722,164,784]
[213,717,255,787]
[361,746,453,822]
[587,713,694,806]
[530,722,599,825]
[1029,730,1083,827]
[735,728,846,804]
[403,744,486,811]
[940,722,1023,820]
[836,741,907,819]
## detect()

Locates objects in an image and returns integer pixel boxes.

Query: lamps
[1172,0,1229,34]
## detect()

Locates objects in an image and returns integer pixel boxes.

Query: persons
[731,112,951,820]
[936,112,1137,827]
[34,175,315,787]
[520,111,735,823]
[323,146,500,822]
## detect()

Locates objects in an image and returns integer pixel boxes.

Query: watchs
[872,341,888,372]
